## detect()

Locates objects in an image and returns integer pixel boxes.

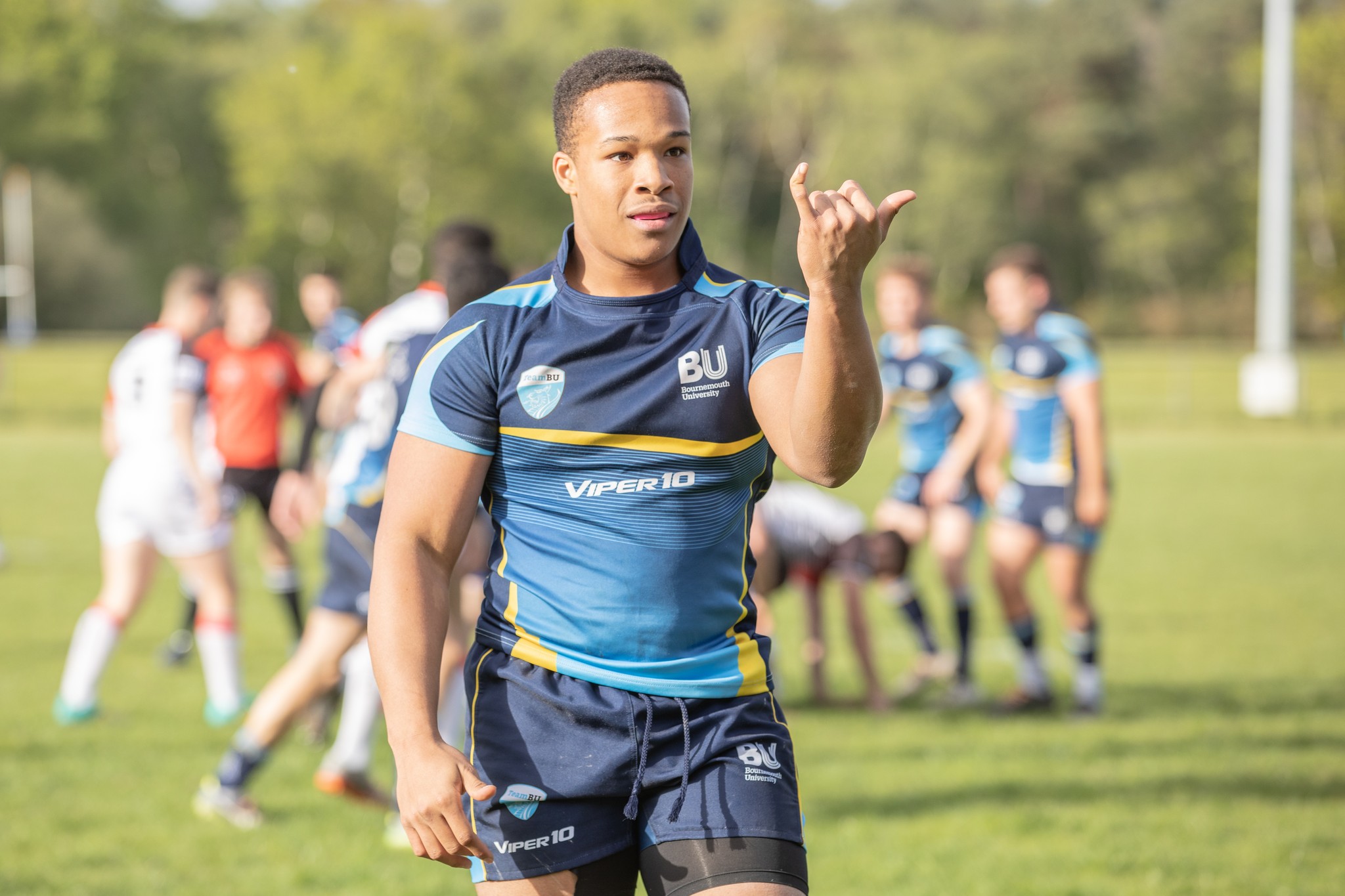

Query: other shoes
[190,776,269,833]
[309,769,400,813]
[200,692,255,726]
[1067,687,1102,722]
[899,676,977,713]
[48,692,100,729]
[983,691,1052,716]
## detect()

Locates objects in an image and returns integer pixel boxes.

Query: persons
[747,242,1108,722]
[366,51,918,896]
[50,220,514,848]
